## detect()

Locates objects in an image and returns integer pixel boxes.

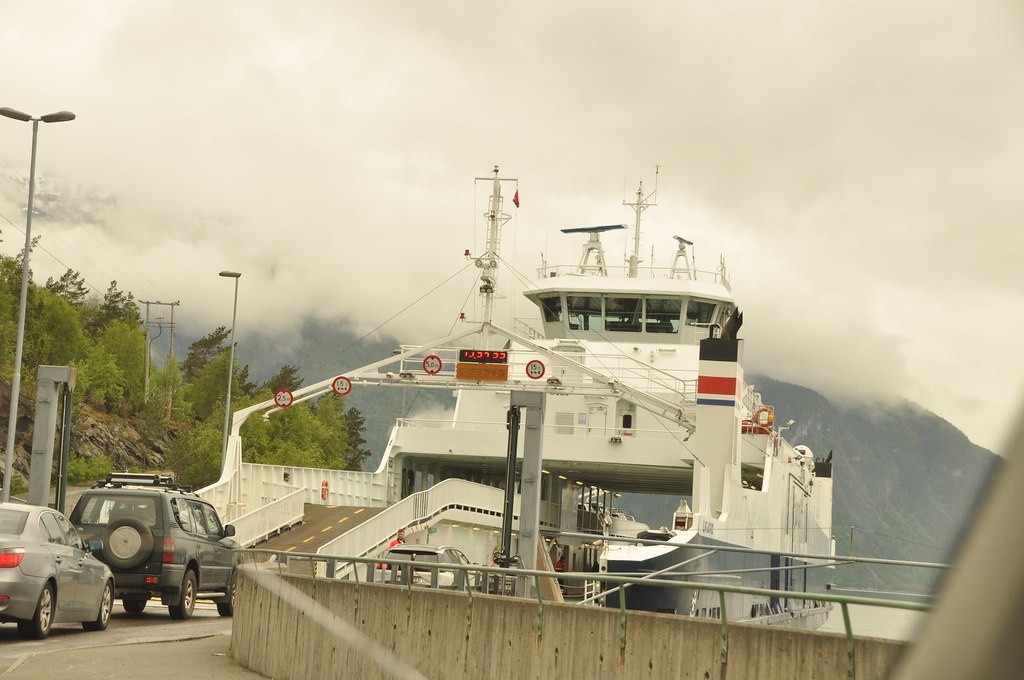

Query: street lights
[215,270,243,480]
[1,105,78,497]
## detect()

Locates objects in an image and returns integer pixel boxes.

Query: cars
[0,502,117,642]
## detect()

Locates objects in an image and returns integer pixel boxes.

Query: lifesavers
[755,408,774,427]
[321,480,329,500]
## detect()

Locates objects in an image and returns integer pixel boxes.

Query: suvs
[375,542,482,588]
[69,472,243,621]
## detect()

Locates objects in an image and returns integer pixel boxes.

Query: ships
[186,158,838,629]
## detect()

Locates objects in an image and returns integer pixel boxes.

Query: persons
[388,529,406,553]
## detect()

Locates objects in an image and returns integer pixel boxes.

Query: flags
[512,189,520,207]
